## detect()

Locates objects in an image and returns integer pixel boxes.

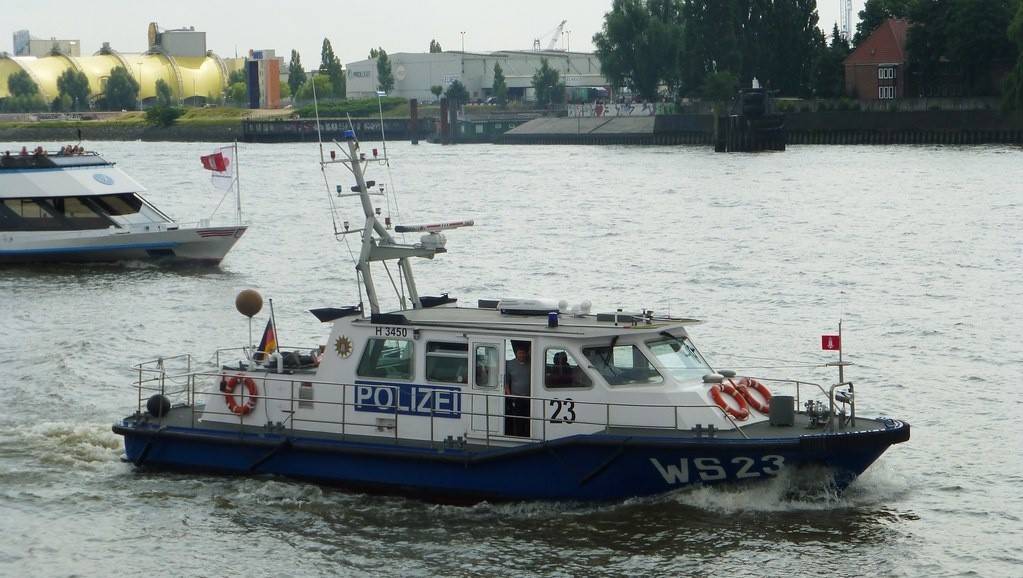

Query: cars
[202,104,219,108]
[484,96,504,105]
[462,98,481,105]
[417,100,439,105]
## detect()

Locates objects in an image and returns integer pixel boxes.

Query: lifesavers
[737,377,773,413]
[712,383,749,419]
[225,373,258,415]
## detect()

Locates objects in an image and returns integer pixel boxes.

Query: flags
[201,152,227,171]
[210,145,233,192]
[253,318,276,360]
[822,335,839,350]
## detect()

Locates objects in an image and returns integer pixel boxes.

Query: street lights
[460,31,466,83]
[562,32,564,50]
[565,31,572,73]
[549,86,552,105]
[136,62,144,111]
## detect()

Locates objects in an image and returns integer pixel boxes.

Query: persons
[77,128,83,141]
[3,151,15,160]
[32,147,43,161]
[58,145,84,157]
[19,146,29,156]
[504,347,531,435]
[591,350,622,385]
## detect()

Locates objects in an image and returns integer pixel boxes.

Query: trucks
[565,88,608,104]
[616,85,646,103]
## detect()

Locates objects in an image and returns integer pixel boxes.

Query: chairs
[553,352,572,386]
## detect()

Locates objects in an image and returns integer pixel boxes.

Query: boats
[0,150,249,269]
[112,62,912,512]
